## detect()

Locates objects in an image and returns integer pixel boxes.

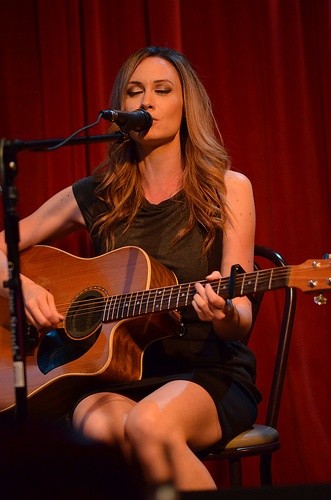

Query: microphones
[100,108,152,132]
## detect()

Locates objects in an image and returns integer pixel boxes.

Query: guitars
[0,244,331,414]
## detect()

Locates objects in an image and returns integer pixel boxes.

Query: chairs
[197,244,297,490]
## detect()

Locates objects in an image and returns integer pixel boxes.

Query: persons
[2,47,257,491]
